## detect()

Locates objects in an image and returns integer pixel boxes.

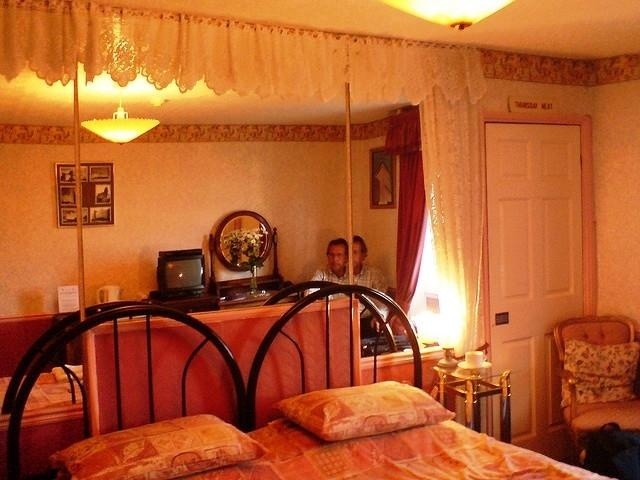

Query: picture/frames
[368,143,400,210]
[52,159,115,228]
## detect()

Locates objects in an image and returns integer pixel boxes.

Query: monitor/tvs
[157,254,207,300]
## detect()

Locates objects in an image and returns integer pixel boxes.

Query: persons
[303,238,349,301]
[353,236,390,339]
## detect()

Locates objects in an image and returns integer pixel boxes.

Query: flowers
[222,231,247,259]
[239,230,265,270]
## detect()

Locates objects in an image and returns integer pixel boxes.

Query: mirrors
[207,210,285,291]
[1,0,480,424]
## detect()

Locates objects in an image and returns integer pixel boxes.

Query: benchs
[0,313,59,377]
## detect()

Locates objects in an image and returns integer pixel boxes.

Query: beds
[4,278,634,480]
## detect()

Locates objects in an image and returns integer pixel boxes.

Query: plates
[458,360,492,369]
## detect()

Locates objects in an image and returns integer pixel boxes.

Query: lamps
[81,75,163,143]
[432,306,465,370]
[383,0,518,33]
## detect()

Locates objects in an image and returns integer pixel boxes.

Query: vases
[248,262,261,296]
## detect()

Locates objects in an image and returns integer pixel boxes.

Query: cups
[464,351,487,365]
[95,285,120,303]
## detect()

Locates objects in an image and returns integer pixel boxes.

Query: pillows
[42,403,269,480]
[555,336,639,411]
[274,369,461,444]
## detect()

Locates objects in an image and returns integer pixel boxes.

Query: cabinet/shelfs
[214,278,298,307]
[146,295,217,313]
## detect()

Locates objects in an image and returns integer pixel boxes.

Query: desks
[0,368,80,419]
[435,364,514,443]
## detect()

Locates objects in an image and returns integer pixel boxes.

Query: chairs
[547,308,639,465]
